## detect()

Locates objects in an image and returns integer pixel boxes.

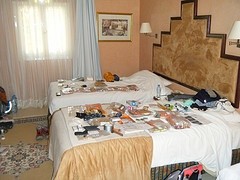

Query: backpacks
[190,88,222,111]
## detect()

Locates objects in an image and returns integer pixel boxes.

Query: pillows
[128,69,172,87]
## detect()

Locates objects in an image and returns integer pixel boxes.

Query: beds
[48,70,240,180]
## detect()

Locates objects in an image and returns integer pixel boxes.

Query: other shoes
[36,125,49,140]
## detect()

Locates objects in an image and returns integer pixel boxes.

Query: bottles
[156,85,161,98]
[11,95,17,112]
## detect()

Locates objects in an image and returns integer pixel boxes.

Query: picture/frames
[96,12,133,41]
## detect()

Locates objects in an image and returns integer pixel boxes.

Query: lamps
[140,22,157,39]
[228,21,240,48]
